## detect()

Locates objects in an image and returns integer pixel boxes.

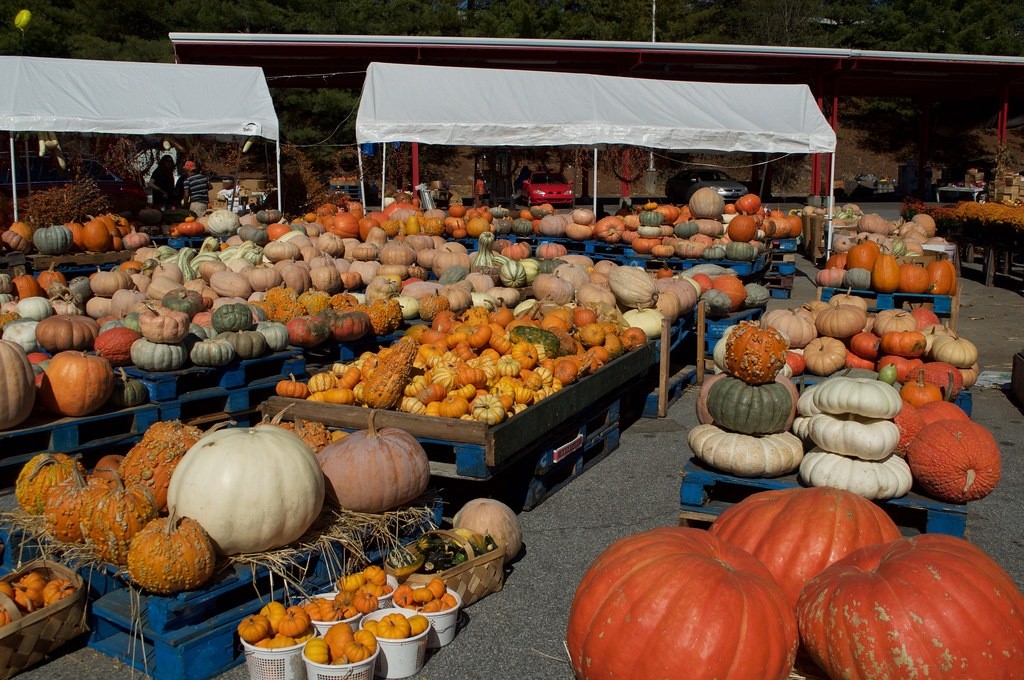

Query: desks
[935,186,983,203]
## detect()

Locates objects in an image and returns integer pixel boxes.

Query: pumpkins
[0,184,1024,680]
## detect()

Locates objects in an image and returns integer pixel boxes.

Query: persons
[149,155,213,217]
[438,180,463,207]
[216,177,250,213]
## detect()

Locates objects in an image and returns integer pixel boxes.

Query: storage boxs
[964,169,1024,203]
[208,179,267,213]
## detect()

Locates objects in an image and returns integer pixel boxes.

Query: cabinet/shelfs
[330,180,361,204]
[0,232,973,680]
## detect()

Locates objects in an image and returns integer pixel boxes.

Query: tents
[356,61,836,260]
[0,56,280,222]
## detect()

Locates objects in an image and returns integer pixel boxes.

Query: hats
[183,161,196,171]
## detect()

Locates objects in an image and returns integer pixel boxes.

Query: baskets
[392,585,461,648]
[297,593,364,635]
[0,558,88,679]
[239,624,317,680]
[358,608,431,679]
[332,572,399,608]
[301,636,380,680]
[384,527,506,609]
